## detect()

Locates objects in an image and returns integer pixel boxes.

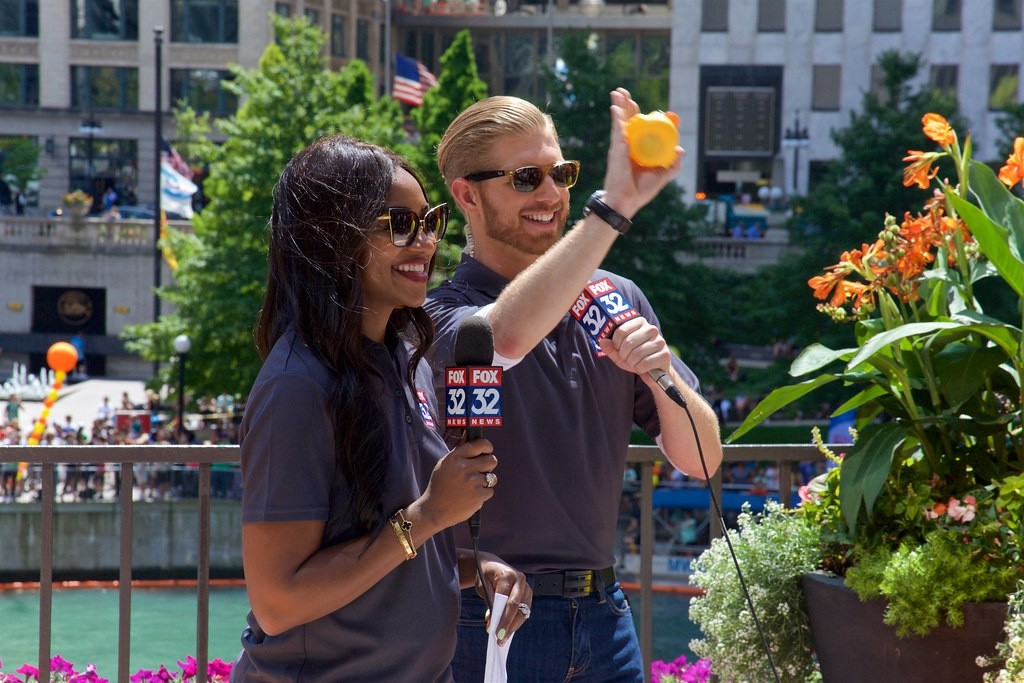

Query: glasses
[371,202,450,246]
[463,158,580,193]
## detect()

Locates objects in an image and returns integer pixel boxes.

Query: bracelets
[395,508,418,559]
[388,516,414,561]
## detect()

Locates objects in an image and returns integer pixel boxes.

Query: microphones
[443,315,503,540]
[569,278,691,409]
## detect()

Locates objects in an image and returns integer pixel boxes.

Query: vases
[792,564,1009,680]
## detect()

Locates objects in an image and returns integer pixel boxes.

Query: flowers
[689,426,1024,682]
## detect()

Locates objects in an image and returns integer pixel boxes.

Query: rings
[485,472,493,487]
[518,602,531,619]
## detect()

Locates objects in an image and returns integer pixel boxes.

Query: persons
[228,133,534,683]
[420,85,727,683]
[0,363,248,507]
[1,169,145,243]
[618,328,860,558]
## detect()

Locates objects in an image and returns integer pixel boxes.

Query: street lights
[783,107,811,189]
[172,333,195,438]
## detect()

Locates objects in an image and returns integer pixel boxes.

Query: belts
[523,569,621,600]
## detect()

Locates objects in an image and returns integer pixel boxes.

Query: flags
[157,142,197,220]
[393,52,440,106]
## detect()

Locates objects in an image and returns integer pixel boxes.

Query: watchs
[581,188,633,235]
